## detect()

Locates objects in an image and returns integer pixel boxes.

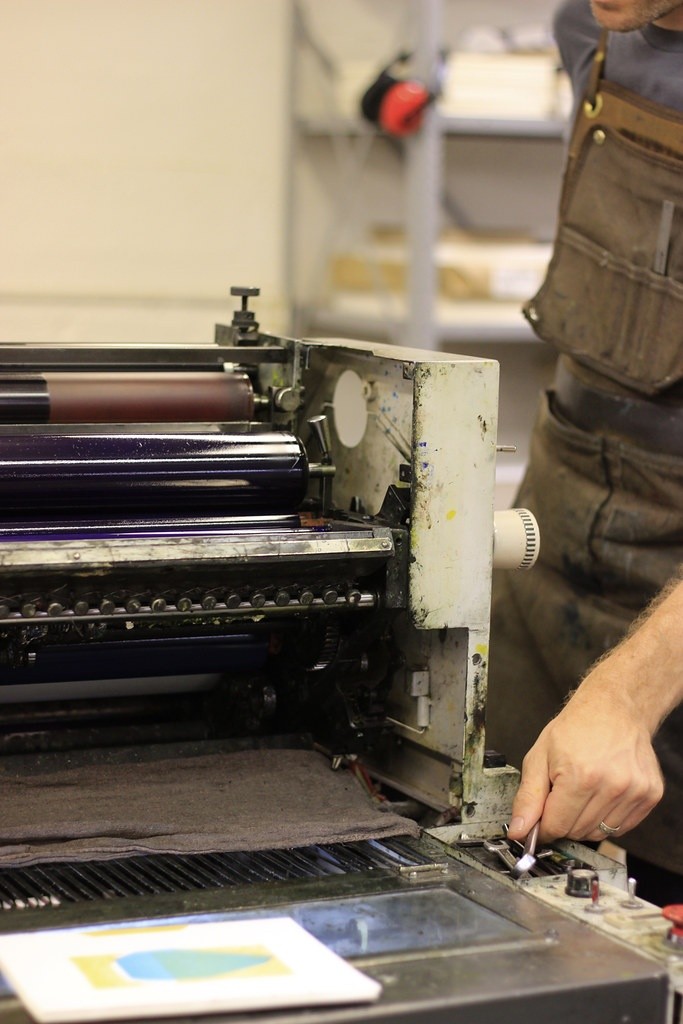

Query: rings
[599,821,620,834]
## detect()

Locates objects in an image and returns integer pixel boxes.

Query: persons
[483,1,682,914]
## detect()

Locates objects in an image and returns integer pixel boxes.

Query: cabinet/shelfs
[282,3,569,514]
